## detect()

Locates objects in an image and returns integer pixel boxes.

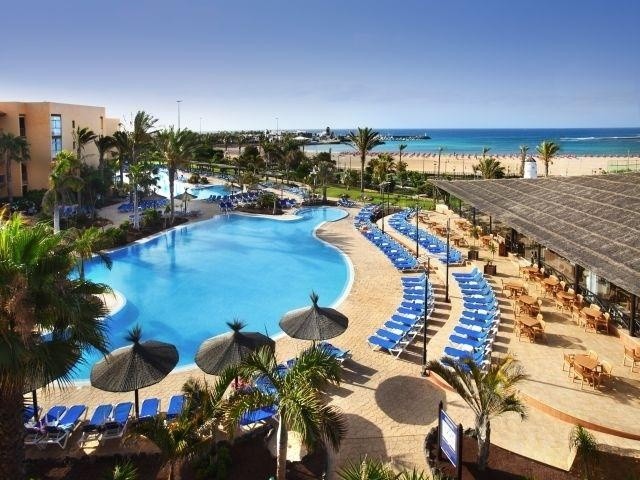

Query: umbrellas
[90,332,178,416]
[140,193,166,210]
[20,341,81,422]
[279,292,348,351]
[195,323,275,389]
[173,190,198,216]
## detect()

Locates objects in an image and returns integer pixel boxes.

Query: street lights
[372,143,453,380]
[199,117,203,133]
[175,99,182,129]
[274,117,279,140]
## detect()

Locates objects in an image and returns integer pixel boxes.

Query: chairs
[319,342,351,368]
[138,397,162,438]
[23,405,67,445]
[119,197,168,213]
[337,198,640,393]
[101,401,134,442]
[166,395,187,421]
[208,189,268,214]
[286,358,297,368]
[40,404,88,449]
[26,404,42,423]
[81,404,110,442]
[277,197,297,209]
[255,374,278,396]
[276,363,287,376]
[240,404,277,425]
